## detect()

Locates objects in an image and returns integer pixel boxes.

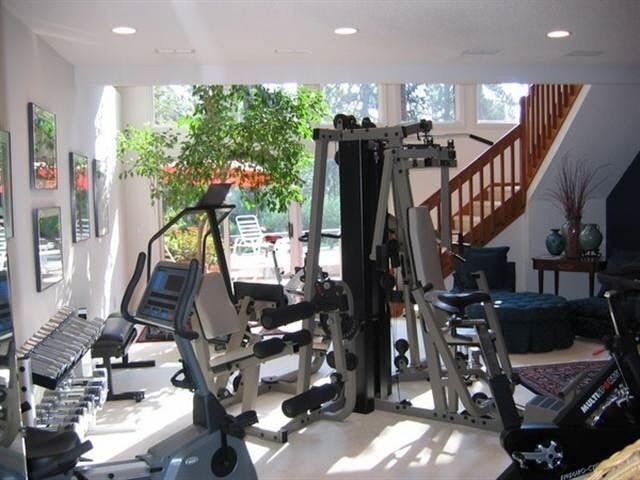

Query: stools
[463,292,574,353]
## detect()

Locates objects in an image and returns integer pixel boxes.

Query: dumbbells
[15,305,107,443]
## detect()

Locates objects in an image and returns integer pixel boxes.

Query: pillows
[455,247,510,289]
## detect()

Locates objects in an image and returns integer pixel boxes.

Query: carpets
[514,361,613,397]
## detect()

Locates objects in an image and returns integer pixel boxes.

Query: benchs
[91,312,155,401]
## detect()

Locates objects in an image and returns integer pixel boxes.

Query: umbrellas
[161,159,283,271]
[0,161,91,193]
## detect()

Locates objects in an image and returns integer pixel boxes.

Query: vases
[578,224,602,258]
[545,228,566,255]
[561,217,585,258]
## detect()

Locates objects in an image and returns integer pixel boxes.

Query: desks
[532,258,607,297]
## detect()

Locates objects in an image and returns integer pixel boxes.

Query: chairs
[572,255,640,338]
[232,214,271,275]
[449,262,516,293]
